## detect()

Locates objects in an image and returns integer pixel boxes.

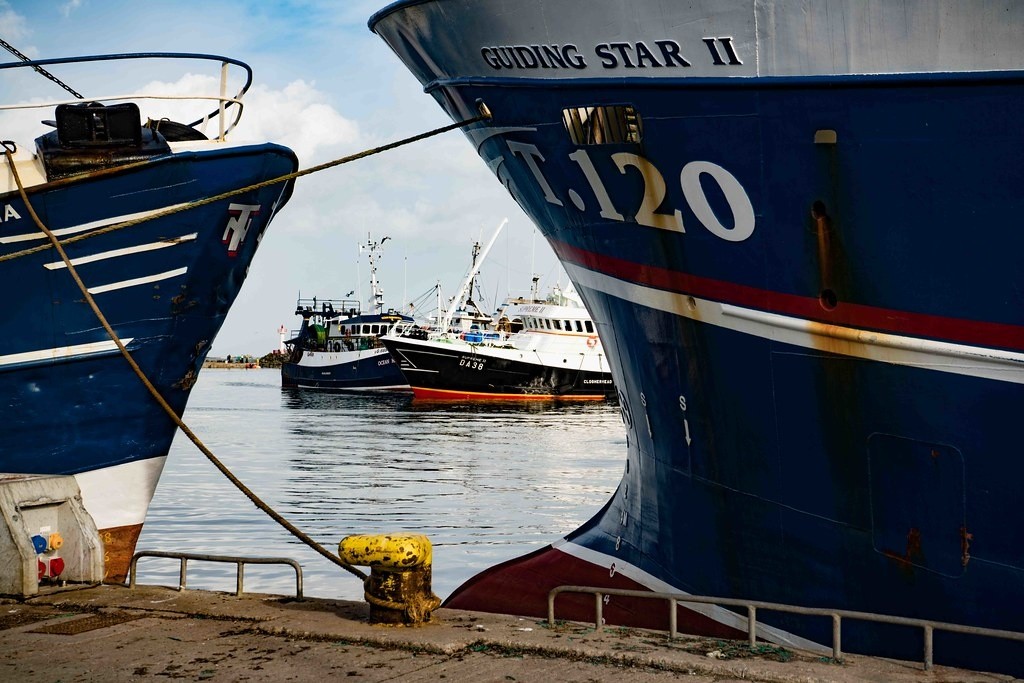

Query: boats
[378,219,618,408]
[0,52,298,588]
[280,232,498,396]
[368,2,1023,680]
